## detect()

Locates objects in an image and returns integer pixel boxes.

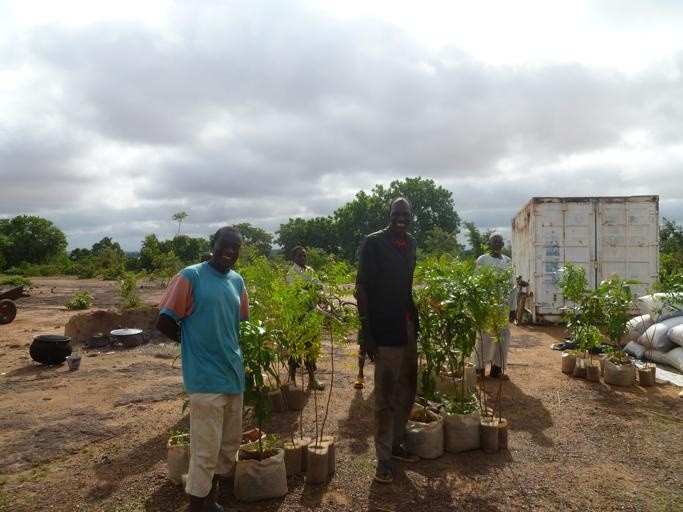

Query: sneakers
[190,495,225,512]
[308,380,325,389]
[390,446,420,463]
[375,459,394,482]
[490,366,509,380]
[353,376,365,389]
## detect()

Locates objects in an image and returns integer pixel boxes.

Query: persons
[468,232,519,382]
[351,269,369,390]
[354,195,422,487]
[283,245,327,392]
[153,224,252,512]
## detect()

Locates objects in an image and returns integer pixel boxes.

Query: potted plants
[397,253,519,460]
[555,262,683,386]
[167,256,363,502]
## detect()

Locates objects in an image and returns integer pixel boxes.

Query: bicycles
[512,271,529,325]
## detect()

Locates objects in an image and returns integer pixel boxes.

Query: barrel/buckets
[66,356,80,370]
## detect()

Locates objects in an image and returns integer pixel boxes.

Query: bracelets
[359,314,368,321]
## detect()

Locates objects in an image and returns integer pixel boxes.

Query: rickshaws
[0,281,33,323]
